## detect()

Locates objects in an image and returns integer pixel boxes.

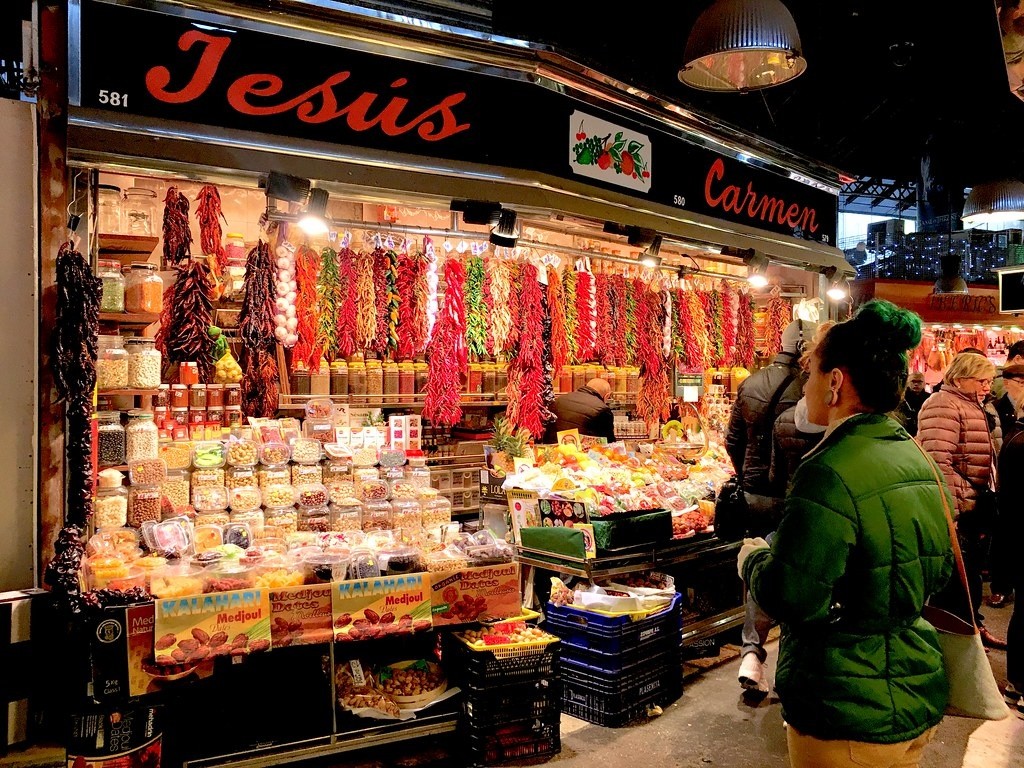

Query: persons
[737,300,959,768]
[934,340,1024,606]
[725,319,809,692]
[543,378,617,444]
[899,372,932,436]
[995,400,1024,719]
[918,352,1007,652]
[766,321,836,490]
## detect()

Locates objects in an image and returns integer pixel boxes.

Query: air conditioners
[866,219,905,250]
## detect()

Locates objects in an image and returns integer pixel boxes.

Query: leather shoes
[985,646,990,653]
[979,627,1008,652]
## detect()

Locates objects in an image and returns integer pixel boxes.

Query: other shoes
[1016,694,1024,719]
[1003,683,1023,704]
[986,592,1008,608]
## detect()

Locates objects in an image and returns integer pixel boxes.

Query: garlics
[275,246,298,347]
[423,250,439,348]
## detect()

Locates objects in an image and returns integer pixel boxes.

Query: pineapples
[491,417,527,471]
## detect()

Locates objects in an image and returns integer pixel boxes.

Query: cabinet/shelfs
[92,233,638,516]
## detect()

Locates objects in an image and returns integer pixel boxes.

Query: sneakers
[737,652,769,693]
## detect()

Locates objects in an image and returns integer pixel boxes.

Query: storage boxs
[431,407,684,768]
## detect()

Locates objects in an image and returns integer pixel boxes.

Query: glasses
[972,379,995,388]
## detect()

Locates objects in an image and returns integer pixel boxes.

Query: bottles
[95,183,158,239]
[94,334,751,542]
[95,258,163,315]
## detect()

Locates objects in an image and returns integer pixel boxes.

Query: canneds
[154,383,241,430]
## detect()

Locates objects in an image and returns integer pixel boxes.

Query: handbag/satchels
[920,605,1010,720]
[714,479,750,542]
[956,483,998,559]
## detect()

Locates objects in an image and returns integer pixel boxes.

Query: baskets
[543,591,682,726]
[450,627,560,765]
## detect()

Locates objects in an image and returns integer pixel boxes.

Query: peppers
[44,527,155,615]
[420,315,467,427]
[441,256,792,441]
[291,246,432,376]
[54,242,100,528]
[155,186,282,423]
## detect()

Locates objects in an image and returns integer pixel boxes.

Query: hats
[1001,364,1024,379]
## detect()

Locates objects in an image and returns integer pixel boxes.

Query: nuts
[155,594,488,667]
[94,422,451,532]
[97,350,162,391]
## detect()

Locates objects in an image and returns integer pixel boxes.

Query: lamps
[625,225,663,268]
[678,0,807,94]
[264,171,331,235]
[743,248,770,287]
[462,198,520,248]
[960,180,1024,231]
[934,210,970,295]
[819,265,846,300]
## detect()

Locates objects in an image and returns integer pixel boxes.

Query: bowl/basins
[659,443,704,455]
[83,515,515,598]
